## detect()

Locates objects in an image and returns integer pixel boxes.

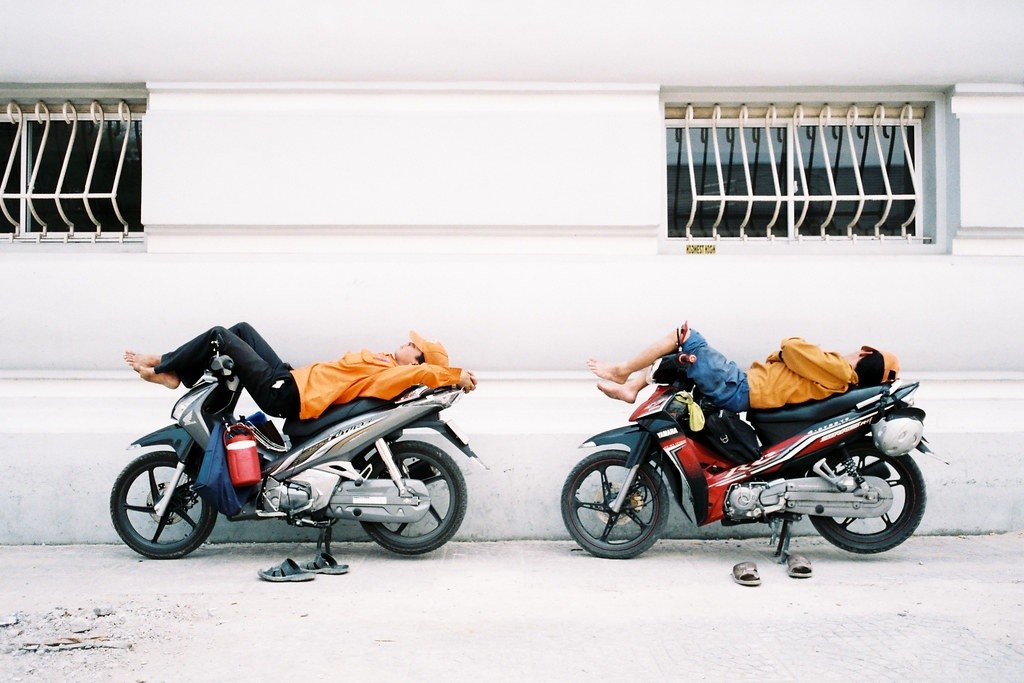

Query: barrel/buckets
[224,425,261,487]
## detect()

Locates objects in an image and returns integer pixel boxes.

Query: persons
[123,322,477,423]
[587,323,901,412]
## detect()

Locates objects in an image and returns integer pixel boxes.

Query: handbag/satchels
[693,390,761,465]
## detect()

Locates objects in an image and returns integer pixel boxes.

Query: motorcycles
[560,370,933,565]
[110,327,477,560]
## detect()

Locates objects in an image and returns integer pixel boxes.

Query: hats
[863,344,899,385]
[409,330,449,367]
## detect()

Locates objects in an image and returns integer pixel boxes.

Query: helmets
[873,406,927,457]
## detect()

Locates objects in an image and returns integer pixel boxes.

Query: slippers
[259,557,316,582]
[731,561,761,586]
[788,554,813,578]
[298,552,348,575]
